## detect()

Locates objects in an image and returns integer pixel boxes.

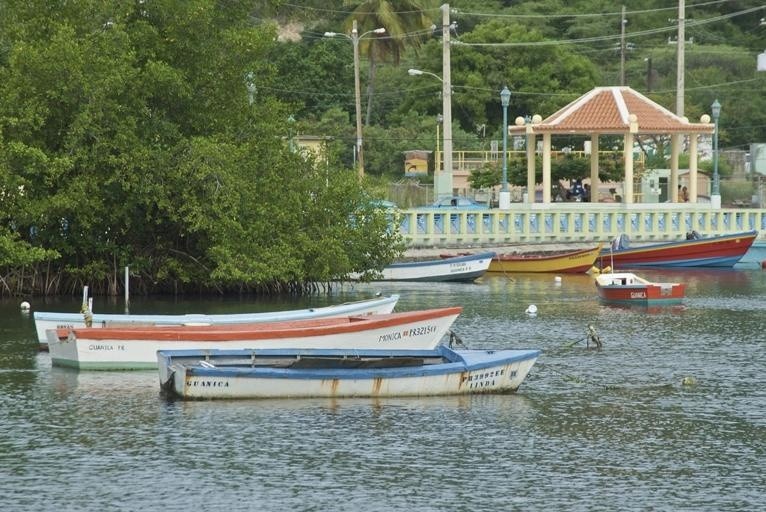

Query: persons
[451,200,456,206]
[563,176,621,202]
[682,186,689,202]
[678,184,685,203]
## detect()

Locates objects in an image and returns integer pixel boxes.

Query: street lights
[323,28,386,183]
[408,68,444,85]
[501,85,511,190]
[711,99,723,195]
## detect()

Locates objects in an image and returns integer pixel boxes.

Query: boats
[441,243,603,274]
[596,230,758,268]
[343,254,494,283]
[595,273,686,307]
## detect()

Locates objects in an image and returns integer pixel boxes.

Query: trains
[403,149,641,179]
